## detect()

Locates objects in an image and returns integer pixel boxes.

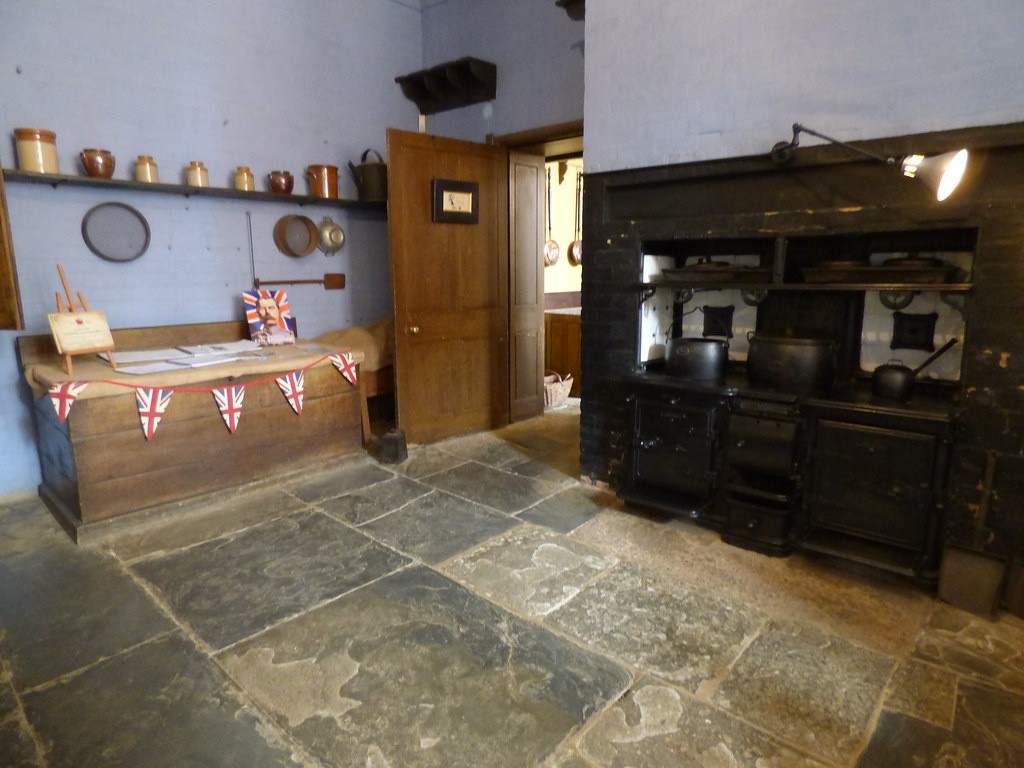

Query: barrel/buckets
[662,306,730,381]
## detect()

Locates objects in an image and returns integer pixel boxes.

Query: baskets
[538,370,573,407]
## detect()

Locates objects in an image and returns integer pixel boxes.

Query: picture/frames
[432,178,479,225]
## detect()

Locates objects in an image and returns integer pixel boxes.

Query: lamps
[772,123,968,202]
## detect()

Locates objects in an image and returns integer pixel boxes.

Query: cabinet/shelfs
[578,121,1024,621]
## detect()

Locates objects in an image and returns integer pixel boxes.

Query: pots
[316,217,345,256]
[745,328,840,396]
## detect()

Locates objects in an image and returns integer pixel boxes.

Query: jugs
[348,149,388,201]
[869,338,958,400]
